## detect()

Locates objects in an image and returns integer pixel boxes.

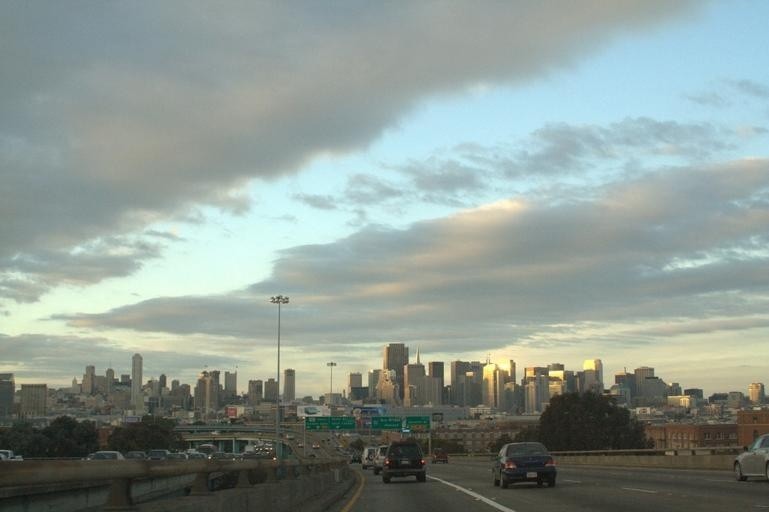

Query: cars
[733,433,769,482]
[667,415,734,426]
[0,439,278,462]
[490,441,557,490]
[211,430,221,436]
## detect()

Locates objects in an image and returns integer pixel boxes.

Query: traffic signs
[405,416,431,433]
[371,416,403,432]
[304,416,330,431]
[329,417,355,430]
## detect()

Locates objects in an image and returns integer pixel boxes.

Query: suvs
[287,432,429,484]
[431,448,449,465]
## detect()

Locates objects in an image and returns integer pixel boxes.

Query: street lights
[268,293,291,459]
[326,360,338,408]
